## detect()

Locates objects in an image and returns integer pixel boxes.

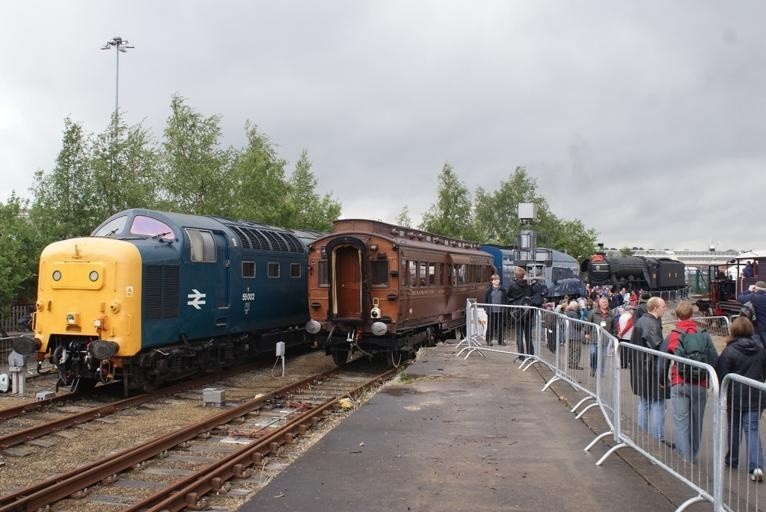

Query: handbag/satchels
[508,295,533,322]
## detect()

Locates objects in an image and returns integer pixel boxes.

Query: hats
[754,281,766,291]
[638,291,652,303]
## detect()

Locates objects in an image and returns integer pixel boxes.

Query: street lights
[103,36,135,143]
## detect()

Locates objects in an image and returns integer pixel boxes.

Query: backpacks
[739,300,757,326]
[671,328,711,382]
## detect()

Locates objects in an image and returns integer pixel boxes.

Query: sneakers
[750,467,763,482]
[486,340,523,364]
[661,440,676,449]
